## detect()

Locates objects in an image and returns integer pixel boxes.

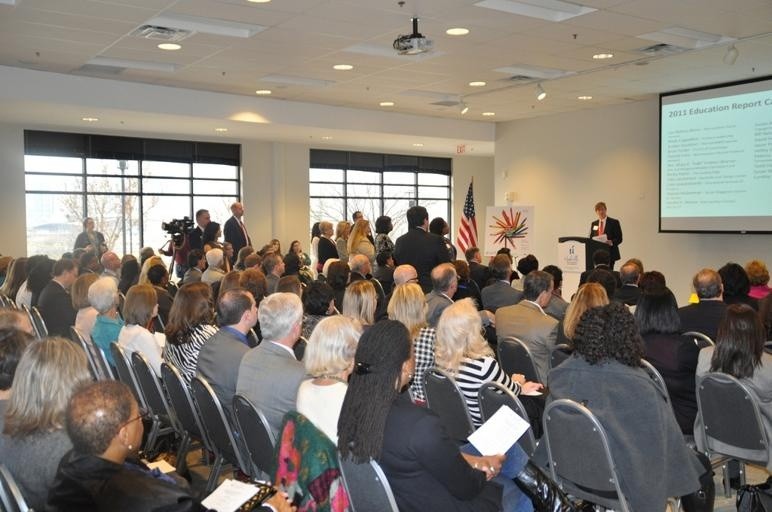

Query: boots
[512,460,596,512]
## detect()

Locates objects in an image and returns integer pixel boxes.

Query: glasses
[406,277,419,283]
[117,407,152,435]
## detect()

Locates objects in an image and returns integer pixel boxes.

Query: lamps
[722,43,738,65]
[536,83,546,101]
[460,97,468,115]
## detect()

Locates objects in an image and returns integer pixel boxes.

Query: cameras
[161,219,194,233]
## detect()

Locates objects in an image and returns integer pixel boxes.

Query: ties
[598,222,603,236]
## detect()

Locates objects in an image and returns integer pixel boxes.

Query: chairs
[291,333,308,362]
[370,277,386,300]
[334,438,400,511]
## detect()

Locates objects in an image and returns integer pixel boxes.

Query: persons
[1,204,771,512]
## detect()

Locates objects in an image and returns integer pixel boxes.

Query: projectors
[392,32,434,58]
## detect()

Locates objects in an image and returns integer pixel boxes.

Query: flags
[457,181,478,255]
